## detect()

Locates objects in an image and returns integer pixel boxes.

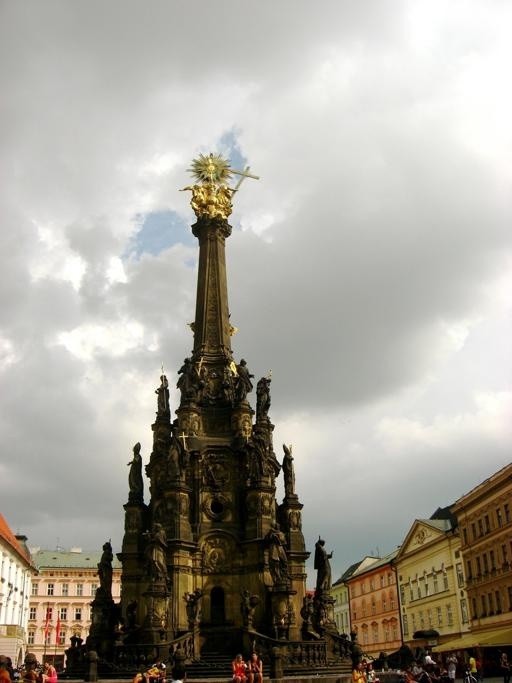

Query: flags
[56,612,60,643]
[45,605,49,639]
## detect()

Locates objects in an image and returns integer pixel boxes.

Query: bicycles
[461,663,479,683]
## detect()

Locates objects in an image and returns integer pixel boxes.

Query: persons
[192,589,201,620]
[127,443,143,501]
[247,652,263,683]
[171,665,187,683]
[232,653,247,683]
[314,540,332,590]
[283,444,294,496]
[352,650,511,683]
[155,376,169,416]
[241,590,250,615]
[268,523,289,580]
[256,378,271,420]
[133,659,167,683]
[235,359,254,400]
[0,655,58,683]
[176,359,195,393]
[144,522,168,584]
[97,543,113,599]
